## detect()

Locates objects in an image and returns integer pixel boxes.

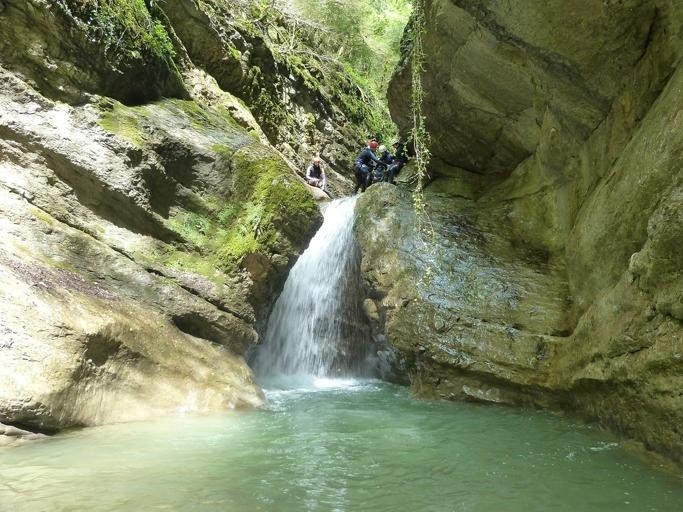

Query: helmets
[313,157,320,162]
[370,138,400,153]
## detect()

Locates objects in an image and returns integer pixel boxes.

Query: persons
[383,139,406,183]
[375,144,391,182]
[305,155,327,192]
[352,138,388,194]
